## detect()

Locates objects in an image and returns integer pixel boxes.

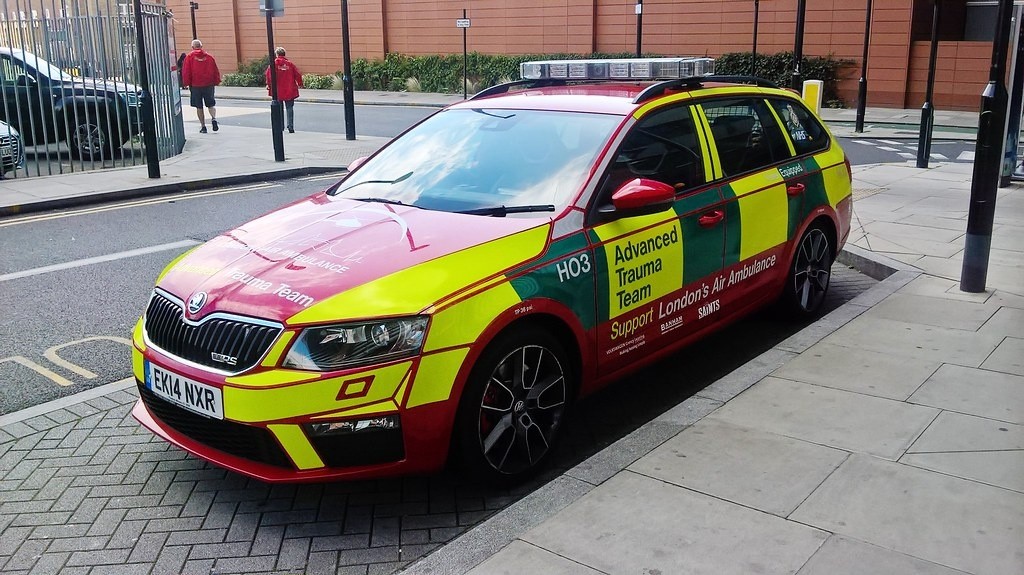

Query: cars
[0,120,26,178]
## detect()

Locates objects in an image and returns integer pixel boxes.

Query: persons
[182,40,220,133]
[265,47,304,133]
[177,53,188,90]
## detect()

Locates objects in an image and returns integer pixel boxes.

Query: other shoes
[200,129,207,133]
[289,130,294,133]
[211,117,218,131]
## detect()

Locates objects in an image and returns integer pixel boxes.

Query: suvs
[0,47,143,162]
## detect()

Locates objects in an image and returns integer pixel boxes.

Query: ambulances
[130,74,854,492]
